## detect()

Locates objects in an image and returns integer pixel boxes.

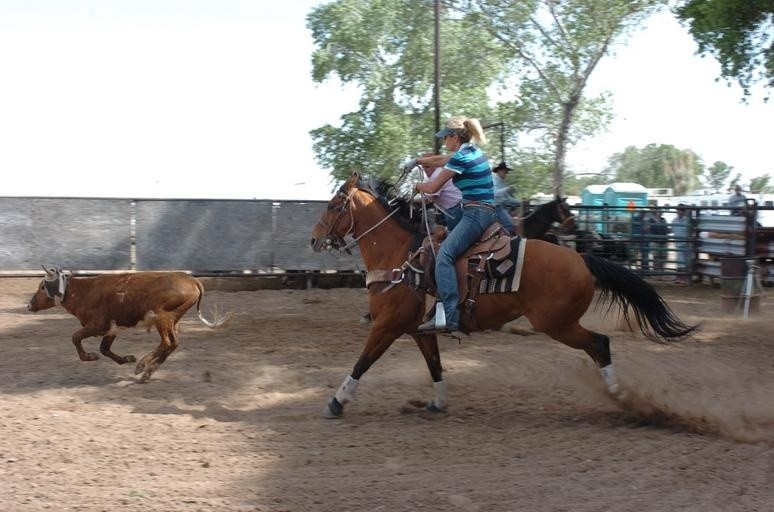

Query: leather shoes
[417,314,458,330]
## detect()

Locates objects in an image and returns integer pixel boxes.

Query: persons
[671,204,692,286]
[399,116,498,332]
[491,162,530,237]
[728,184,750,217]
[647,207,672,273]
[631,210,651,277]
[413,151,462,232]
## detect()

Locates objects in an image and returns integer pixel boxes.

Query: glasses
[443,136,447,139]
[422,165,429,168]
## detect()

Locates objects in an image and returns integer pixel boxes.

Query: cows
[27,264,233,384]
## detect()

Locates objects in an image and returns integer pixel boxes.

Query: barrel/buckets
[719,254,761,314]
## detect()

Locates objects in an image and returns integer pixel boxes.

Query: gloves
[399,179,419,199]
[399,158,417,172]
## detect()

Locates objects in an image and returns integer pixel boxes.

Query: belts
[463,201,496,210]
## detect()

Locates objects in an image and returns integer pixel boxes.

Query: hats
[493,162,513,173]
[435,127,468,138]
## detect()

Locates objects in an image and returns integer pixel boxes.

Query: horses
[310,171,703,418]
